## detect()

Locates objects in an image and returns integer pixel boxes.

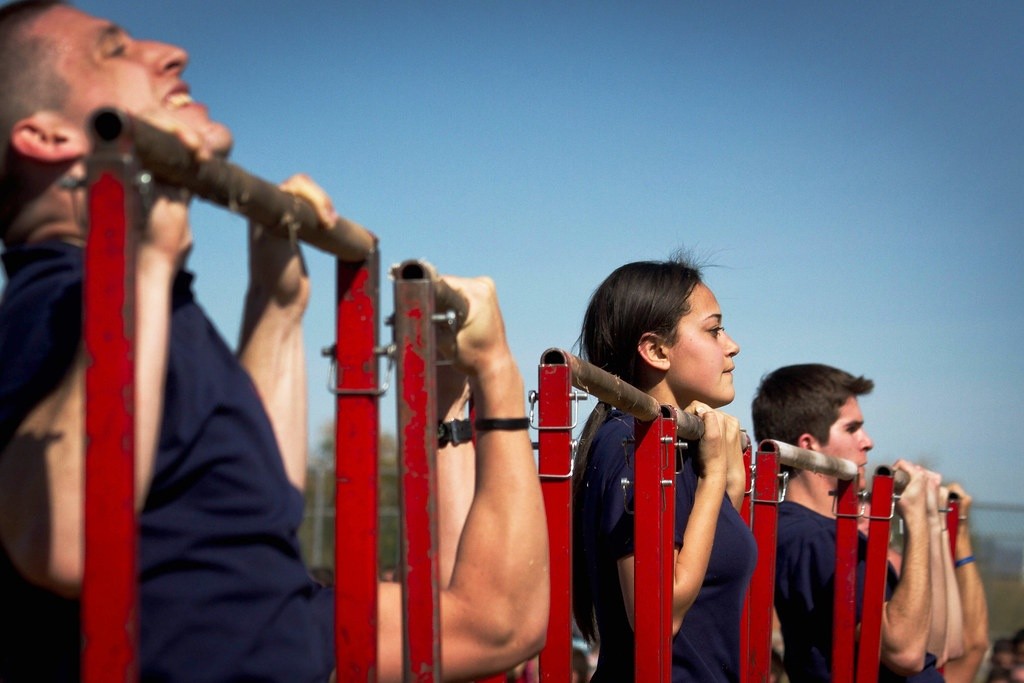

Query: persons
[570,257,759,683]
[752,363,1024,683]
[0,0,551,683]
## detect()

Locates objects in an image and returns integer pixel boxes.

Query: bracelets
[475,418,531,432]
[436,418,472,449]
[955,555,976,566]
